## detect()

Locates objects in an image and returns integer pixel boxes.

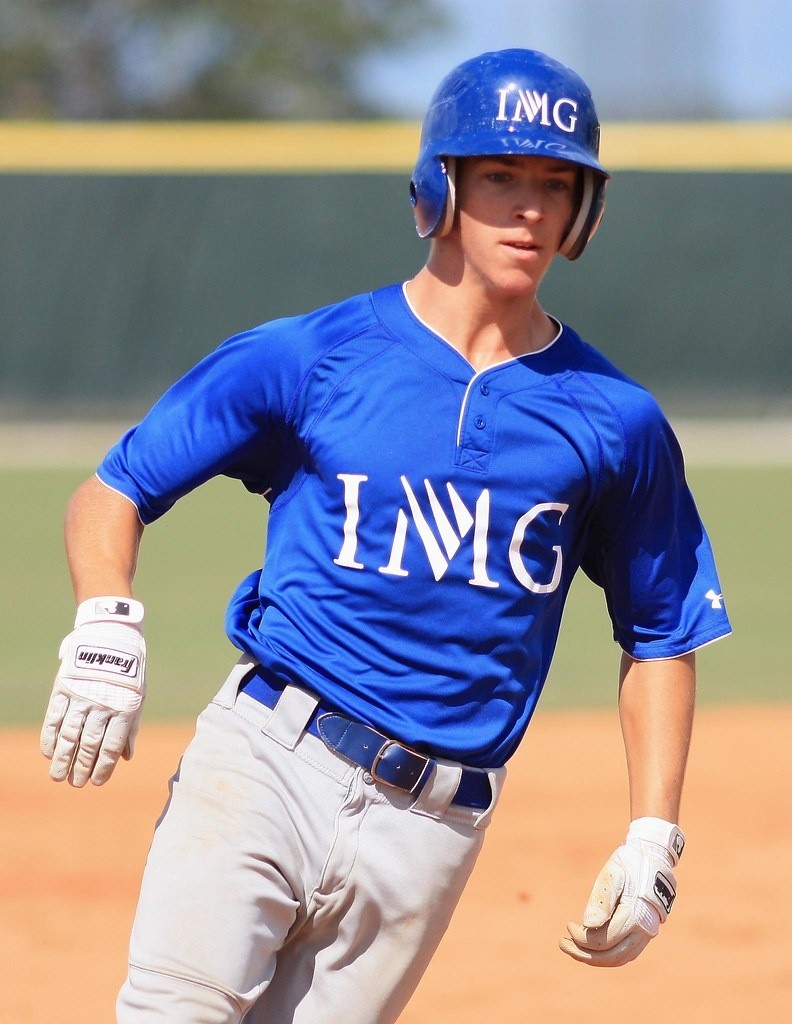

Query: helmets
[409,47,611,261]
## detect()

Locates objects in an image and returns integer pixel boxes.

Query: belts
[240,664,493,809]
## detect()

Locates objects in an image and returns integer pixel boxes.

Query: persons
[41,49,734,1024]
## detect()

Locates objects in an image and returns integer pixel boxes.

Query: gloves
[556,815,685,969]
[42,596,151,788]
